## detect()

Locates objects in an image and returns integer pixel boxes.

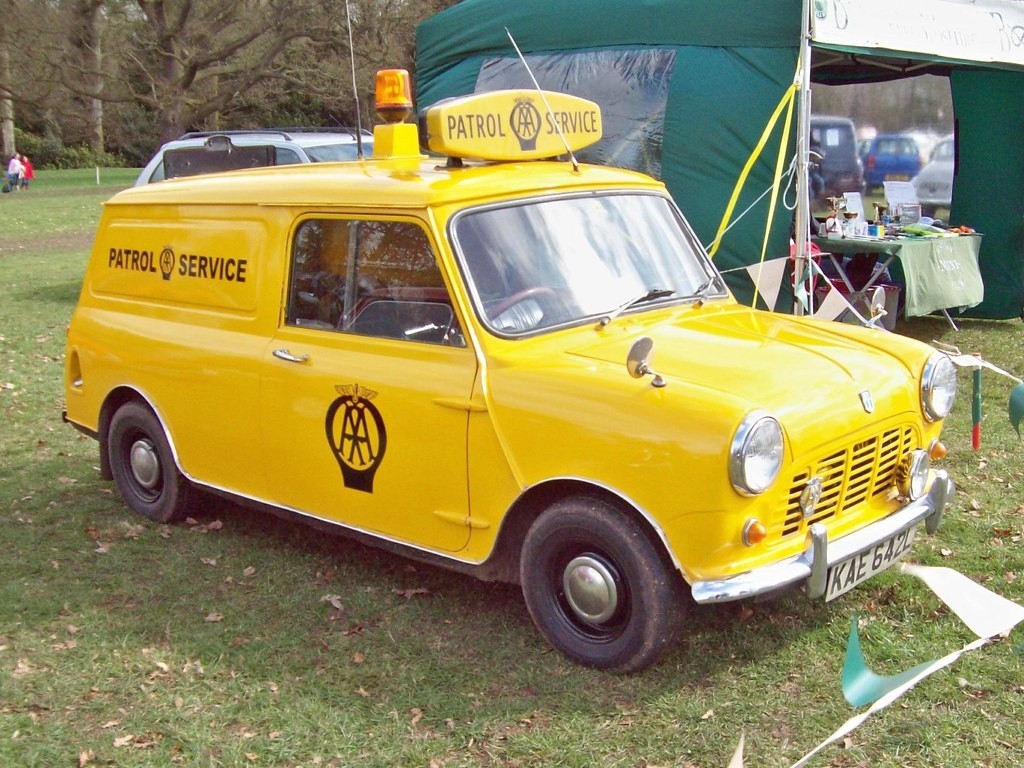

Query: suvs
[136,115,374,186]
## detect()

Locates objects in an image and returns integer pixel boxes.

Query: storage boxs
[815,277,900,333]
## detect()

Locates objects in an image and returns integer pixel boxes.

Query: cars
[62,26,959,675]
[809,116,954,218]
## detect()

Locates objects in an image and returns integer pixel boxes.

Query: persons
[20,155,35,190]
[8,153,21,191]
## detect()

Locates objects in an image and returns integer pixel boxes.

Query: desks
[809,233,985,334]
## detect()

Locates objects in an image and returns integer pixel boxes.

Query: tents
[416,0,1024,328]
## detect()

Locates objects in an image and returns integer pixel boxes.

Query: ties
[503,256,516,290]
